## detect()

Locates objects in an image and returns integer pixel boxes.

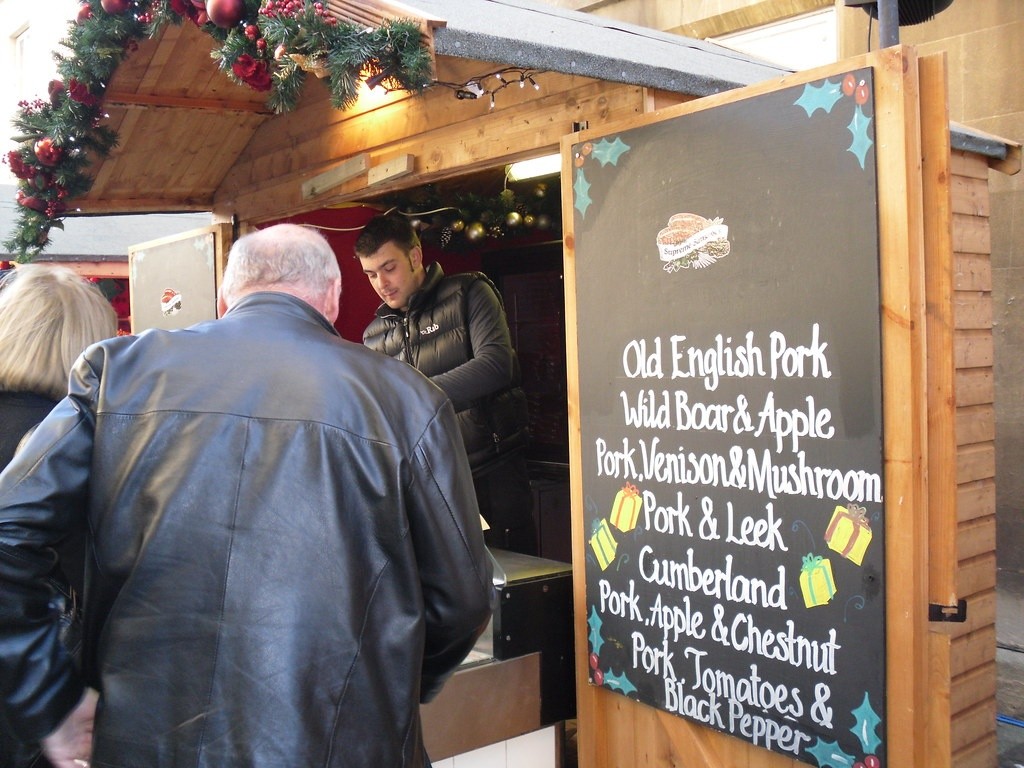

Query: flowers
[0,0,436,281]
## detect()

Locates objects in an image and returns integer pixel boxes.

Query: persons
[1,224,492,768]
[0,265,119,471]
[343,215,537,552]
